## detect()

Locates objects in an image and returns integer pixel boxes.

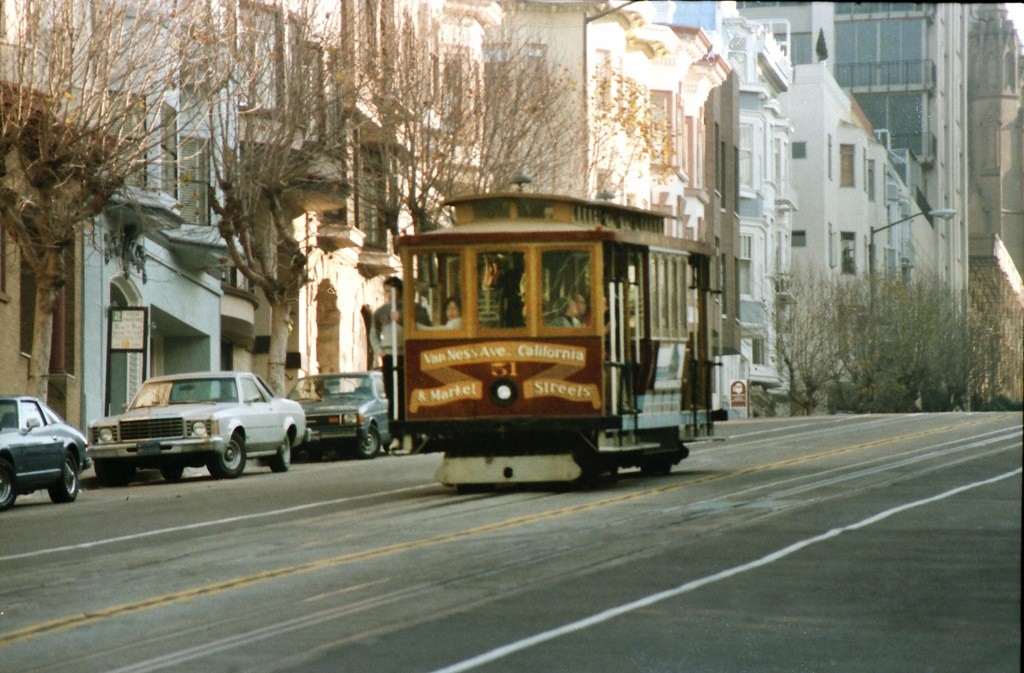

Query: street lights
[869,207,957,364]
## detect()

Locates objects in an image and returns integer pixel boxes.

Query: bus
[391,172,727,488]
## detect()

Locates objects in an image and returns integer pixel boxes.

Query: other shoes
[396,435,412,454]
[389,438,401,450]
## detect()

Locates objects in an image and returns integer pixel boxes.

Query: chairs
[2,412,17,428]
[355,386,371,394]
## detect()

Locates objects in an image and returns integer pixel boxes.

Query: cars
[87,371,308,489]
[286,372,391,459]
[1,395,93,511]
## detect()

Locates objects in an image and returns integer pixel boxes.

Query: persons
[549,294,588,326]
[415,298,462,330]
[367,277,431,456]
